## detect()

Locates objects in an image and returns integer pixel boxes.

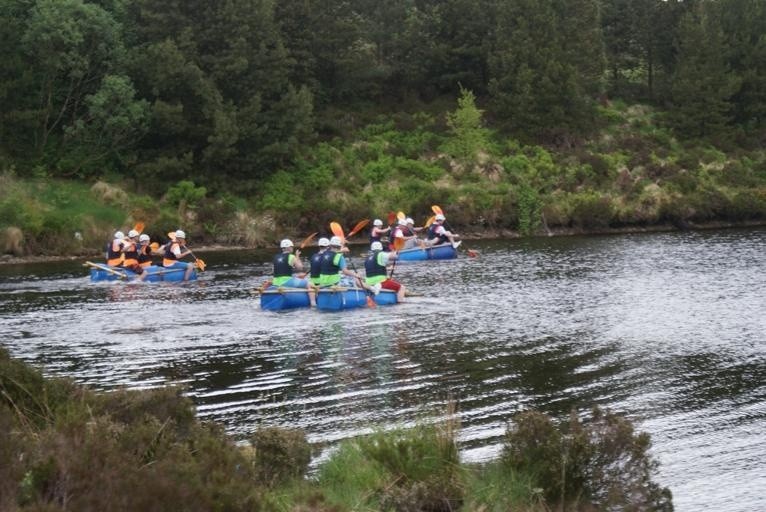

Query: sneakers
[372,283,381,295]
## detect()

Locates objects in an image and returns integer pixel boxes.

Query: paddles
[148,243,159,250]
[134,222,144,235]
[330,221,377,309]
[393,216,435,250]
[432,205,476,257]
[168,232,205,271]
[387,211,396,238]
[263,233,317,290]
[305,220,370,274]
[397,212,426,248]
[389,238,407,279]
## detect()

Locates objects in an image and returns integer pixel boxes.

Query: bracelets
[352,272,356,277]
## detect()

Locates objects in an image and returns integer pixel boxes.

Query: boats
[92,263,197,282]
[261,282,396,311]
[364,243,458,261]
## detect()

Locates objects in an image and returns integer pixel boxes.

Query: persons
[269,236,321,314]
[319,235,382,296]
[104,228,195,283]
[369,214,463,253]
[362,240,410,304]
[308,236,330,287]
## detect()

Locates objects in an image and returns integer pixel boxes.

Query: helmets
[114,229,186,242]
[280,236,384,251]
[373,214,446,226]
